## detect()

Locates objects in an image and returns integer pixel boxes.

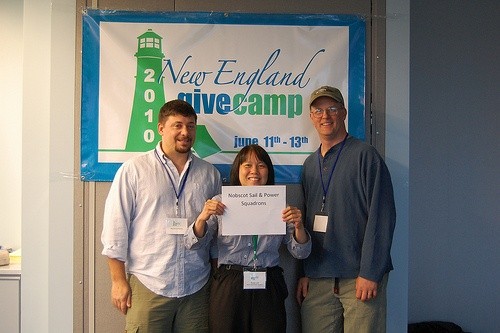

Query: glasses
[311,105,343,117]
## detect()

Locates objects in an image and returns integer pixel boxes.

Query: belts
[219,265,284,273]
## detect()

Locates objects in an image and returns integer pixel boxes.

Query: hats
[309,85,344,105]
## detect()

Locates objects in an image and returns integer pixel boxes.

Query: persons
[101,100,222,333]
[294,85,397,333]
[182,144,312,333]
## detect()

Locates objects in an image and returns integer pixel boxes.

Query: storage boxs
[9,249,21,264]
